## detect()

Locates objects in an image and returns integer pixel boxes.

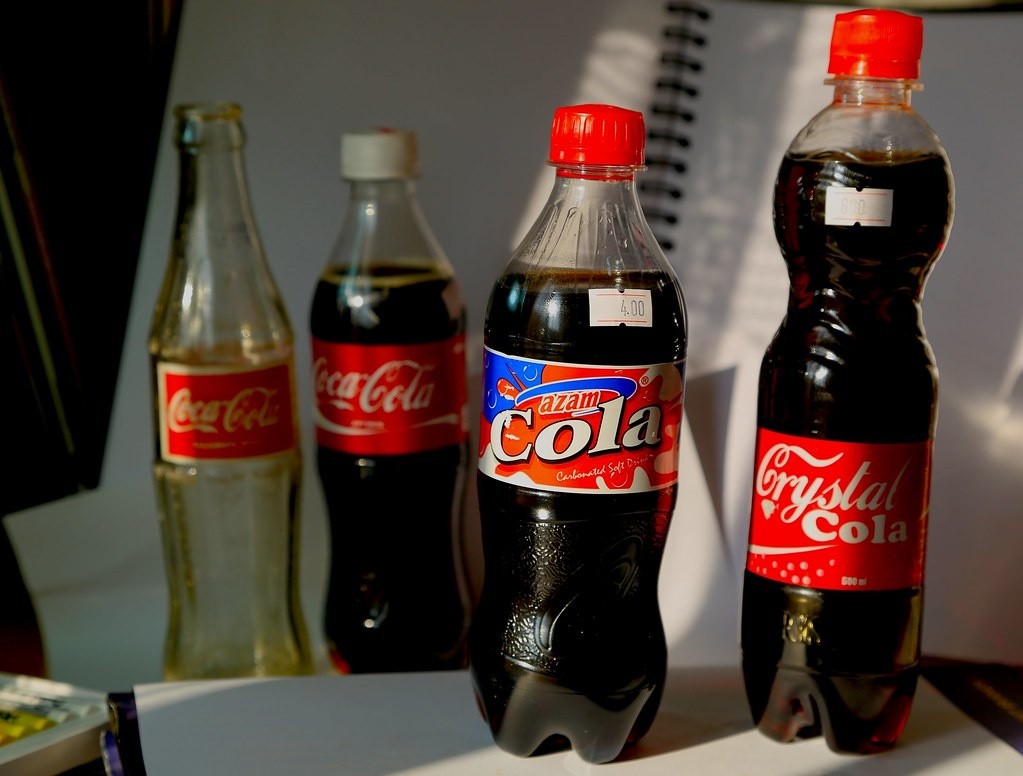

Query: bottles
[148,99,317,680]
[469,102,691,765]
[309,125,474,667]
[738,9,959,758]
